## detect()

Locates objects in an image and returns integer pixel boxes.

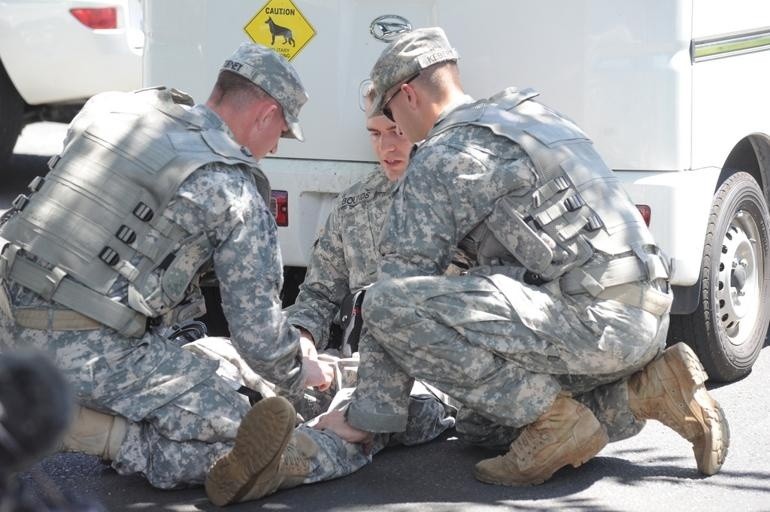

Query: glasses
[378,72,425,123]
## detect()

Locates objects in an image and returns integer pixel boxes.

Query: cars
[0,1,142,165]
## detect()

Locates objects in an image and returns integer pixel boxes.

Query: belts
[0,285,105,334]
[596,282,677,318]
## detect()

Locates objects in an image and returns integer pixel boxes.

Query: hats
[218,39,310,144]
[364,26,461,122]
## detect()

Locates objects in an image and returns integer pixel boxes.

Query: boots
[470,390,613,488]
[202,394,311,509]
[626,339,731,478]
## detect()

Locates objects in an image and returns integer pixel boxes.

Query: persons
[0,42,335,448]
[312,26,733,488]
[46,78,477,510]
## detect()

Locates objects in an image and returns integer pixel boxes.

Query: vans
[142,0,769,381]
